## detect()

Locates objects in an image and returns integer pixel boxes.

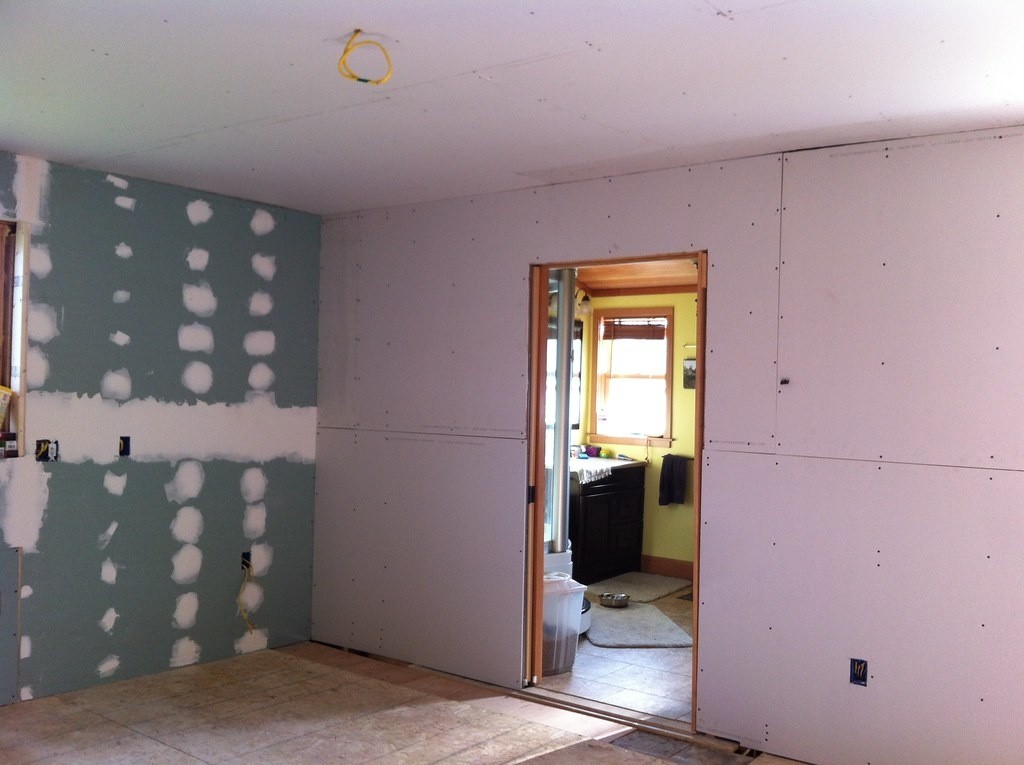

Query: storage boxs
[542,569,587,677]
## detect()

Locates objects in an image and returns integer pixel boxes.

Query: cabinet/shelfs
[568,455,649,586]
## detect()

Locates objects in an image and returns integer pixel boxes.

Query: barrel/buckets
[542,571,587,677]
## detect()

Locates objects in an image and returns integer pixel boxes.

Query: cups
[570,445,581,457]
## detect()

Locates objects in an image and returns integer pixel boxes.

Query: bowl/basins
[598,593,630,608]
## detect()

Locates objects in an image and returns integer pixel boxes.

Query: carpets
[584,602,693,649]
[586,572,693,602]
